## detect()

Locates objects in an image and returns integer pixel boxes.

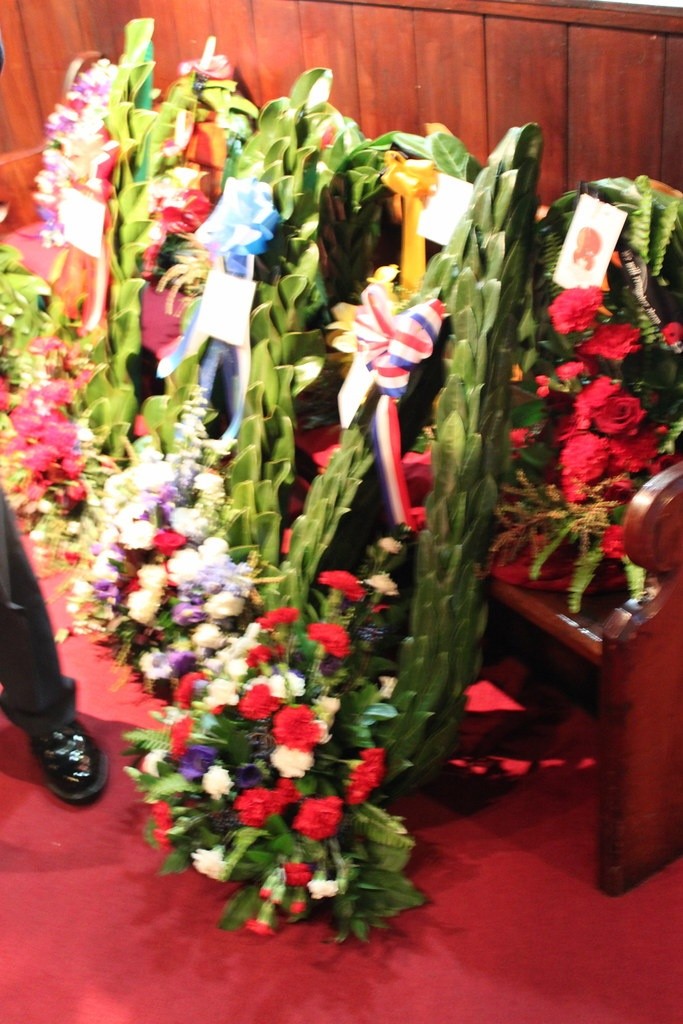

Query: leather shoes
[32,719,109,804]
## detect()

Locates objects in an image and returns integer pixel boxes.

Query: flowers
[0,23,683,943]
